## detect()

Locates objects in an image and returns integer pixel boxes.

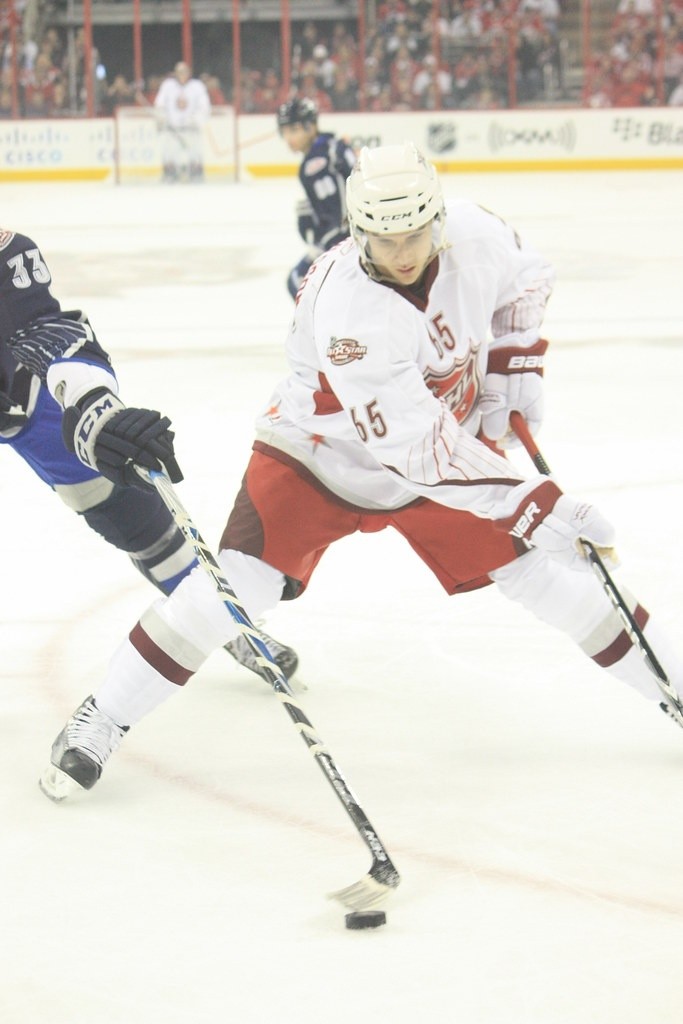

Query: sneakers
[233,632,297,685]
[38,695,127,797]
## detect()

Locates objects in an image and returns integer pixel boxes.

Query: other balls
[344,910,387,931]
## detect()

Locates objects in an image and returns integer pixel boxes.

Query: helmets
[345,142,448,270]
[276,99,318,137]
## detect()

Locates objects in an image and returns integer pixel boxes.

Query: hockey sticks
[137,465,404,913]
[510,408,683,730]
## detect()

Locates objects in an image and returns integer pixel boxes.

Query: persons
[0,225,300,690]
[152,61,220,187]
[277,96,359,300]
[1,0,225,118]
[37,144,682,805]
[235,0,682,109]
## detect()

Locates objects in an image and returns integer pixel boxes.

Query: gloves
[492,476,622,590]
[479,339,553,452]
[62,388,183,500]
